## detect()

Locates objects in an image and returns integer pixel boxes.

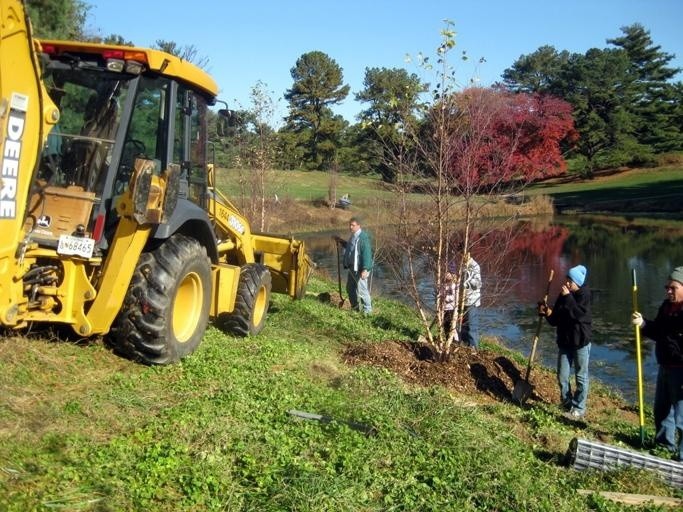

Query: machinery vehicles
[0,0,318,366]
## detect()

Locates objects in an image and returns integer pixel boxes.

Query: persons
[457,244,482,352]
[541,264,599,419]
[338,216,378,321]
[631,266,683,461]
[438,272,457,342]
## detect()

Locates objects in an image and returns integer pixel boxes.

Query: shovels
[337,241,345,309]
[511,269,554,406]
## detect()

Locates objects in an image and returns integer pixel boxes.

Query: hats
[566,265,586,287]
[667,266,682,285]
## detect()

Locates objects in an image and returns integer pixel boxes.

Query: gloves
[536,301,551,318]
[631,312,642,327]
